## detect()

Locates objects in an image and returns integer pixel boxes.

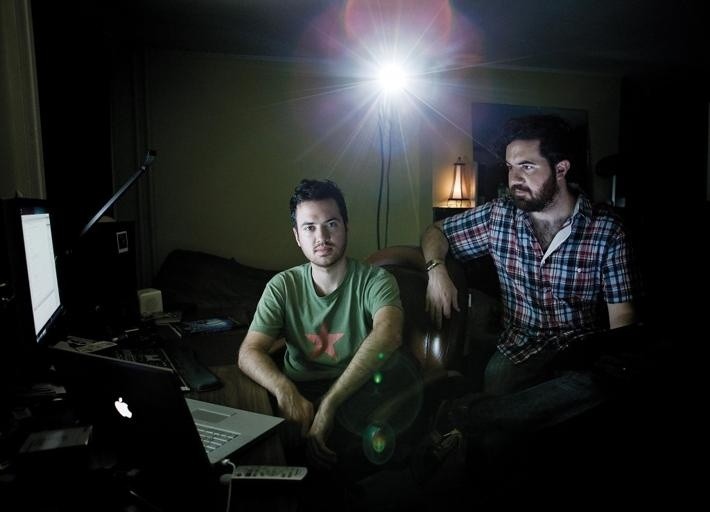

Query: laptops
[46,347,286,470]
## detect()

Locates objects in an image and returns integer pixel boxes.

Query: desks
[1,308,299,511]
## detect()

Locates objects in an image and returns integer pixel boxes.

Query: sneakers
[409,397,459,484]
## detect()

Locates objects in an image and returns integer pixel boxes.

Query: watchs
[423,259,447,271]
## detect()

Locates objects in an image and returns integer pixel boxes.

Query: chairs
[363,247,469,411]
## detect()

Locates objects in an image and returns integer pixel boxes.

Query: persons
[420,112,638,512]
[237,177,419,511]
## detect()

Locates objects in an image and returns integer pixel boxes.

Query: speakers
[137,288,163,315]
[77,220,138,303]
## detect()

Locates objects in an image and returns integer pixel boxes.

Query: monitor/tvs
[15,207,63,344]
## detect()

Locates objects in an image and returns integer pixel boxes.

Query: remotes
[232,465,308,485]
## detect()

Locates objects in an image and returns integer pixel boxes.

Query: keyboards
[130,347,190,392]
